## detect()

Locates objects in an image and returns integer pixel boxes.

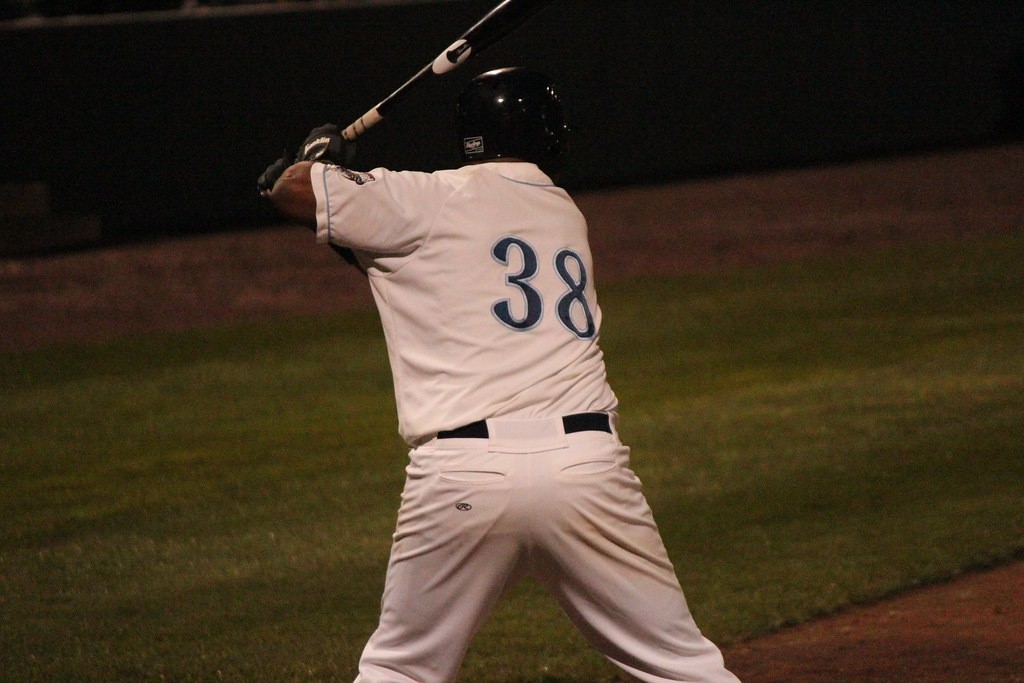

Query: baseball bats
[264,1,525,194]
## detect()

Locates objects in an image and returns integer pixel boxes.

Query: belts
[437,413,612,439]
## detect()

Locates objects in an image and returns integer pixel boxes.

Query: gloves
[257,124,360,192]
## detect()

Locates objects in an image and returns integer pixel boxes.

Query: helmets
[455,68,571,172]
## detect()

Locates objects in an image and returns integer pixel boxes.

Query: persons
[272,66,741,683]
